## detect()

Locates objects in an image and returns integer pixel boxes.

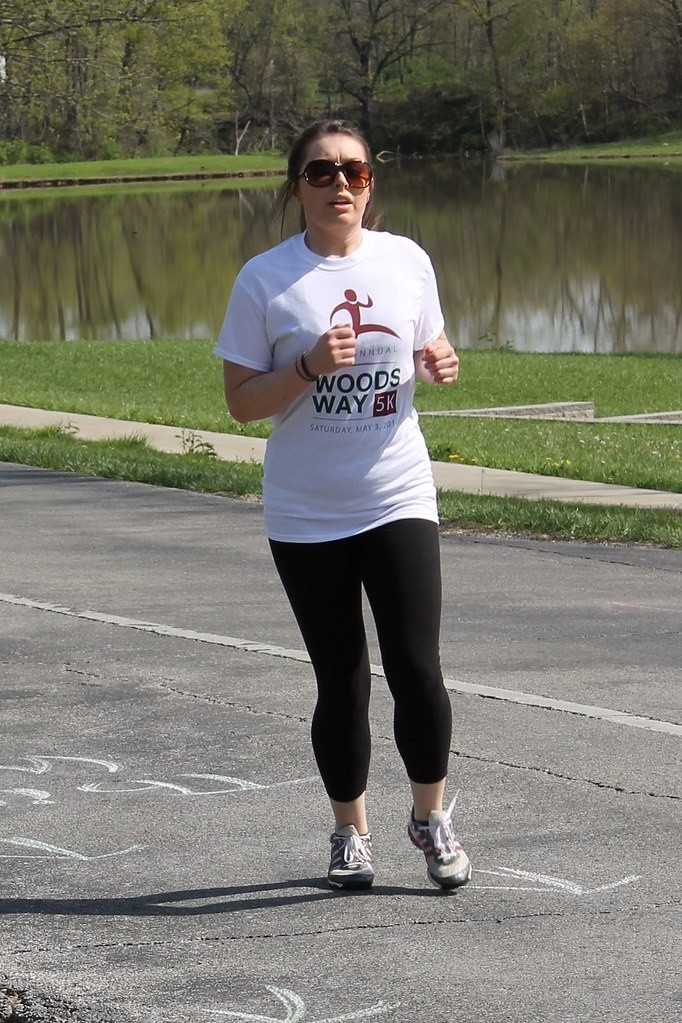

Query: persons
[210,119,470,890]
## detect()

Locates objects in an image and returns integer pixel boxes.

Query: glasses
[296,157,374,187]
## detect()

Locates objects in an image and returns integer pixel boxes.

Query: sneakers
[327,823,376,889]
[407,790,473,890]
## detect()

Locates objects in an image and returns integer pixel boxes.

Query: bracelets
[295,352,319,382]
